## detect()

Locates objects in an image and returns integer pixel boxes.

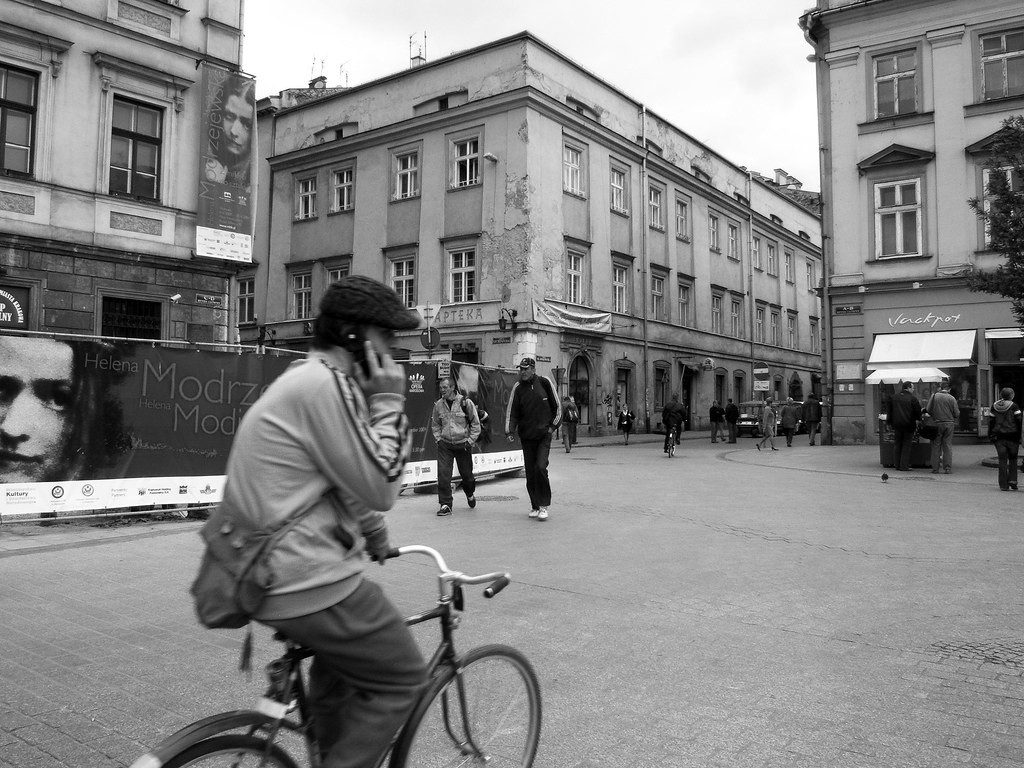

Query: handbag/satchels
[989,433,999,444]
[662,411,670,424]
[186,501,275,630]
[628,419,633,429]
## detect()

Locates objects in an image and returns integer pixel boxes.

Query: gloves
[364,528,390,567]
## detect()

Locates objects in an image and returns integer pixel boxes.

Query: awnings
[866,330,976,370]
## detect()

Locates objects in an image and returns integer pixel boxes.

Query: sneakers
[528,508,539,518]
[437,504,452,516]
[537,506,548,520]
[467,496,476,508]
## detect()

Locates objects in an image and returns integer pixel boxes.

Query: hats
[318,274,419,329]
[515,357,536,368]
[1001,387,1013,400]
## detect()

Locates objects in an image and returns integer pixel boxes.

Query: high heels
[756,443,761,451]
[772,447,778,450]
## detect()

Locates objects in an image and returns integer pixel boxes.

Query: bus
[733,399,823,437]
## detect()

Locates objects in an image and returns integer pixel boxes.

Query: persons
[617,403,635,444]
[222,275,431,768]
[800,392,822,445]
[433,378,481,515]
[987,387,1021,491]
[717,401,726,441]
[662,393,686,453]
[710,400,720,443]
[724,399,739,443]
[561,397,579,453]
[781,397,799,447]
[755,397,778,451]
[504,358,562,521]
[926,382,960,474]
[0,337,128,483]
[209,74,257,194]
[886,381,922,470]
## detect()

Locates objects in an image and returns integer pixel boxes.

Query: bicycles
[667,417,688,459]
[123,544,543,768]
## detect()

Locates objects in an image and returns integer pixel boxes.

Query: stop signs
[753,360,770,381]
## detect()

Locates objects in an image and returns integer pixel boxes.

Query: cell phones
[341,322,384,382]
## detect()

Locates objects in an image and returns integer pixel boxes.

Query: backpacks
[564,406,578,423]
[460,396,492,444]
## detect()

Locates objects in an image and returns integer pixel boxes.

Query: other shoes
[945,469,952,473]
[895,467,913,471]
[677,438,680,445]
[665,446,668,452]
[932,470,940,473]
[1009,482,1019,490]
[566,445,572,453]
